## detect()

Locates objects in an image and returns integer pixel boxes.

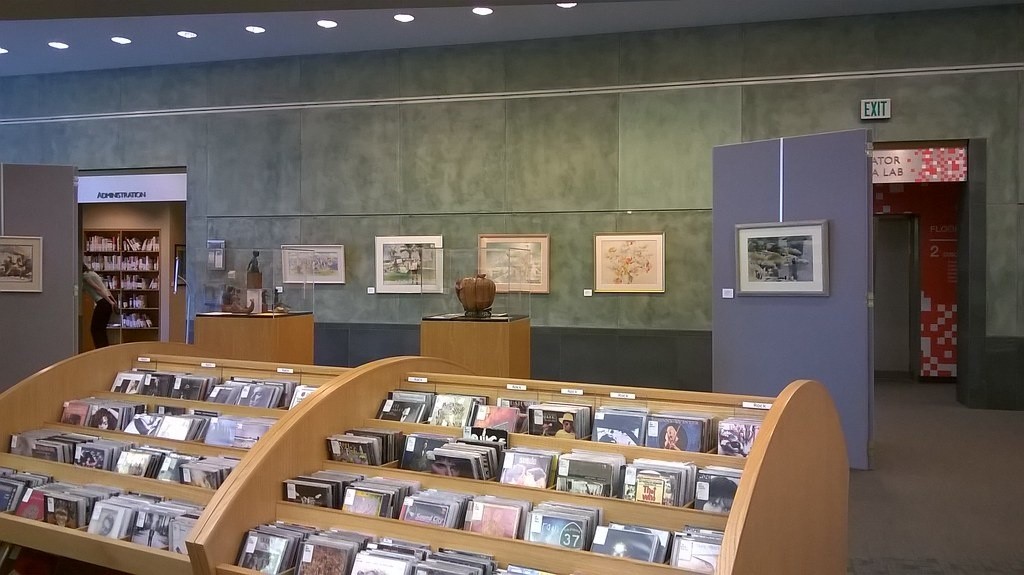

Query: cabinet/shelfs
[0,341,851,575]
[81,228,161,344]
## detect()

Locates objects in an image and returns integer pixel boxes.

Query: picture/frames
[0,235,43,293]
[593,231,666,293]
[281,244,346,285]
[174,244,186,286]
[478,233,550,294]
[734,219,830,298]
[374,234,444,294]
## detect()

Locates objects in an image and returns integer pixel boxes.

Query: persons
[431,456,463,477]
[542,413,575,439]
[99,514,114,537]
[247,250,260,271]
[262,289,268,303]
[53,503,72,528]
[147,527,154,546]
[82,263,117,350]
[90,408,115,430]
[274,288,279,304]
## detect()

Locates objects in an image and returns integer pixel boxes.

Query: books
[0,233,768,575]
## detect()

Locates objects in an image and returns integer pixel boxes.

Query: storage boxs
[195,246,315,318]
[420,245,534,323]
